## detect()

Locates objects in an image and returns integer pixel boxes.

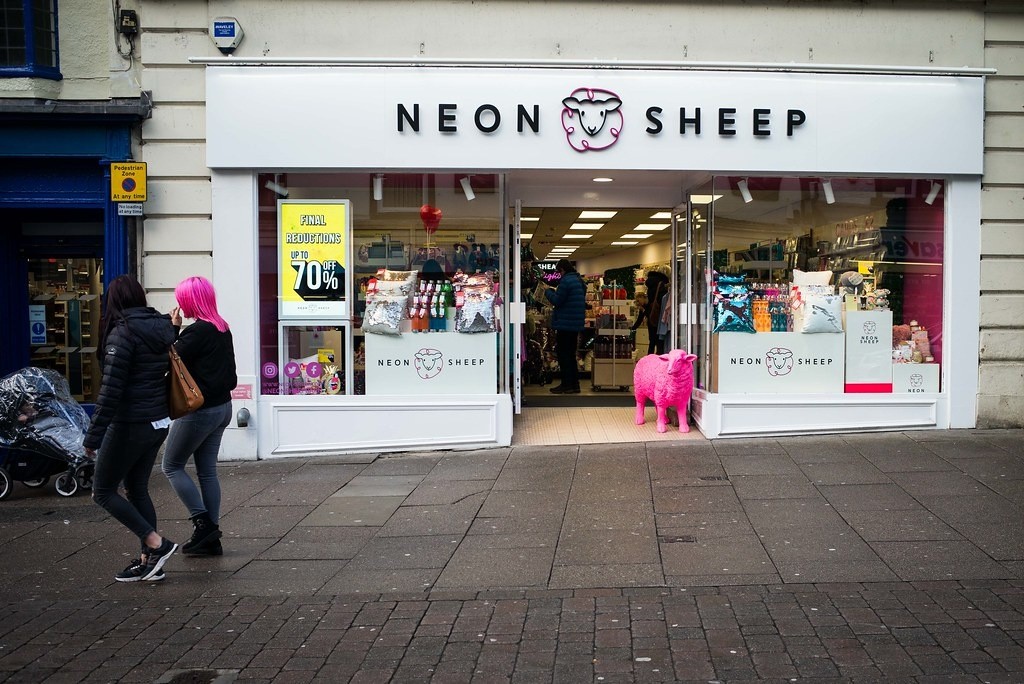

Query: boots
[182,511,223,556]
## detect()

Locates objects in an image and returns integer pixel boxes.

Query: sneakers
[550,383,580,394]
[115,536,179,582]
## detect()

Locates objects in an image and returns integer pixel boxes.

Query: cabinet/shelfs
[783,228,813,282]
[353,235,407,371]
[591,280,636,391]
[743,238,788,283]
[817,226,897,294]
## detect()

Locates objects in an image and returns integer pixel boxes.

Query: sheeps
[633,349,697,433]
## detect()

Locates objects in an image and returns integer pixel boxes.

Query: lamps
[373,174,387,201]
[265,174,288,196]
[925,180,941,205]
[459,175,476,202]
[737,177,753,203]
[819,176,836,204]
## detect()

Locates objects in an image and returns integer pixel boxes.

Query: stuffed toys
[893,324,910,352]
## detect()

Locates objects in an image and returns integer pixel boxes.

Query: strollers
[0,365,98,503]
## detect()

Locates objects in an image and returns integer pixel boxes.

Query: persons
[81,273,179,582]
[420,259,451,283]
[542,259,586,394]
[162,275,238,556]
[630,265,672,356]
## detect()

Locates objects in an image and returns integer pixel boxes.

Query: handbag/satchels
[168,343,204,420]
